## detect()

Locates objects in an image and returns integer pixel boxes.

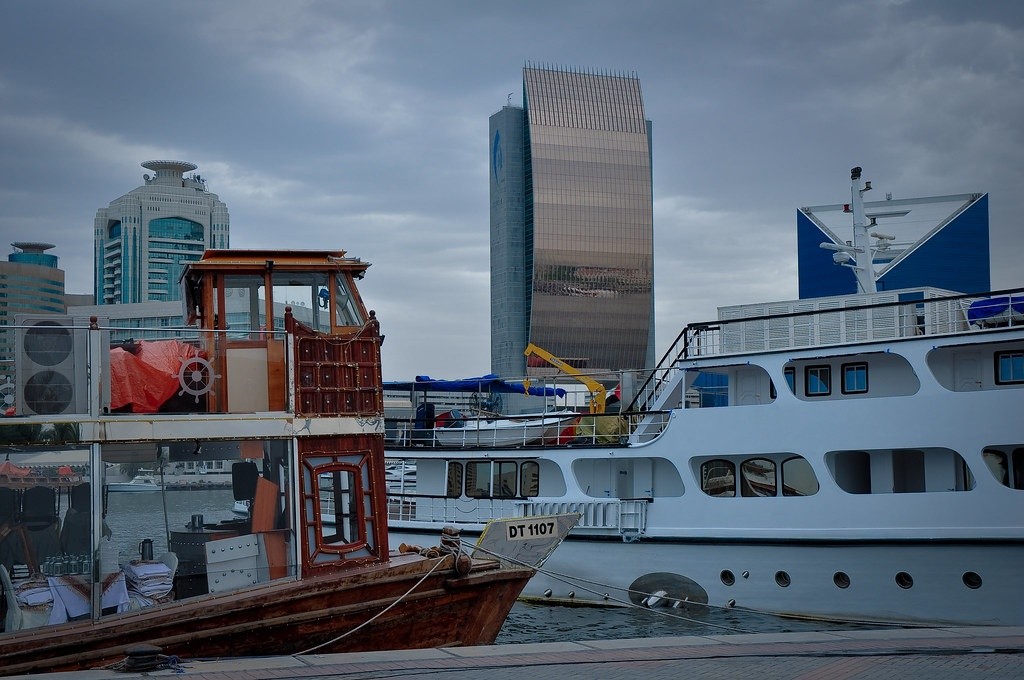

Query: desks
[169,524,241,601]
[46,569,130,627]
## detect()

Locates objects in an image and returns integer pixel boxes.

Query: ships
[302,165,1024,629]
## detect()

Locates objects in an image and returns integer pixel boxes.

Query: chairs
[0,486,179,639]
[0,563,54,632]
[117,551,179,614]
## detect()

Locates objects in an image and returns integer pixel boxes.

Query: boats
[106,468,167,494]
[431,410,582,449]
[0,246,586,680]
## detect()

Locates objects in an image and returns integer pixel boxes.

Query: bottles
[42,554,91,577]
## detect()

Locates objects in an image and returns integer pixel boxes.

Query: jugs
[191,514,204,532]
[139,539,154,563]
